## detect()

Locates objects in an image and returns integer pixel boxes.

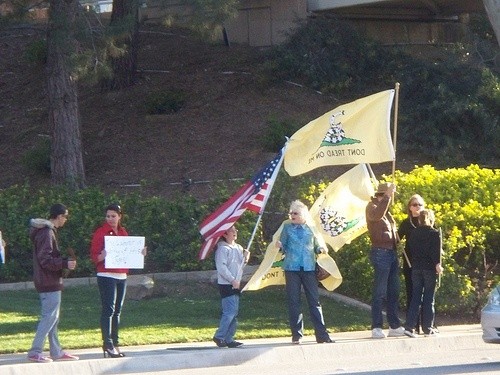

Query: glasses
[230,229,238,232]
[412,203,423,207]
[288,211,298,215]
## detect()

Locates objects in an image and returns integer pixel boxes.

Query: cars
[480,280,500,346]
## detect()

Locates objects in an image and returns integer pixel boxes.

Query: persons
[28,203,79,362]
[90,202,147,358]
[366,182,406,338]
[275,200,335,343]
[213,225,251,348]
[396,195,443,337]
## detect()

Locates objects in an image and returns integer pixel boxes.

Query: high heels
[102,345,125,357]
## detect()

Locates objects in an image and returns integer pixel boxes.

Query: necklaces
[410,217,416,228]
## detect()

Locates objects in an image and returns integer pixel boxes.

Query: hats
[375,182,400,196]
[105,201,122,213]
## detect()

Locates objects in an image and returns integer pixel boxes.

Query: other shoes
[212,336,226,347]
[292,336,299,343]
[424,329,436,336]
[27,354,53,362]
[227,340,243,347]
[387,326,405,336]
[59,354,78,361]
[404,328,416,337]
[372,329,385,337]
[316,338,333,343]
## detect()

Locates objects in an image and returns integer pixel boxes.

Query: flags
[199,150,285,260]
[284,89,394,177]
[309,164,374,252]
[240,220,342,292]
